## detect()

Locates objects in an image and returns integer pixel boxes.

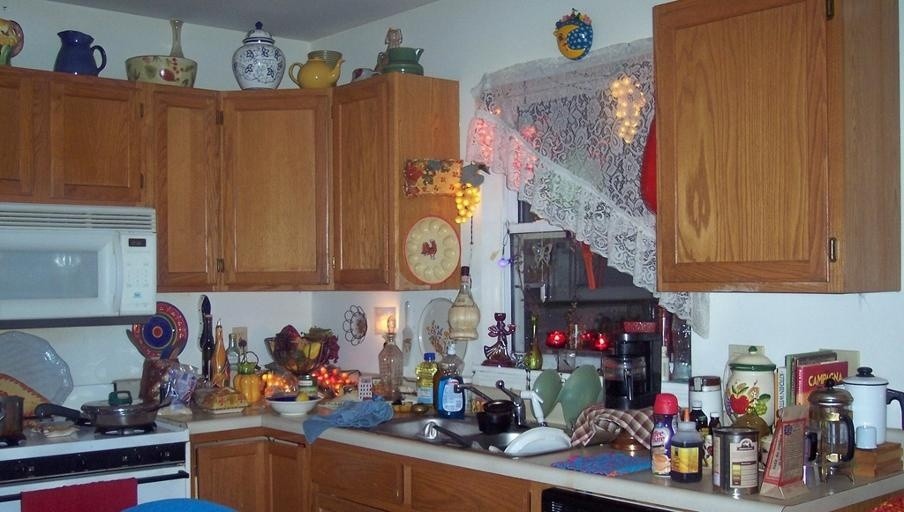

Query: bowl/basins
[550,363,602,425]
[265,393,325,416]
[125,54,198,88]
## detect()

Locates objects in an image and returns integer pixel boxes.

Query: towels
[21,478,137,512]
[302,401,394,445]
[551,451,652,477]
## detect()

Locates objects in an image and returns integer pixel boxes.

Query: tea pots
[287,54,346,88]
[54,29,107,76]
[377,48,427,75]
[831,366,904,447]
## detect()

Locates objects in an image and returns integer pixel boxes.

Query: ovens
[0,463,192,512]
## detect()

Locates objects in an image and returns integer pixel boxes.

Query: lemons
[295,392,309,401]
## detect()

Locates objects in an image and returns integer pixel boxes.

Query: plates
[130,301,188,359]
[528,367,562,422]
[505,426,573,458]
[417,298,468,363]
[0,331,74,419]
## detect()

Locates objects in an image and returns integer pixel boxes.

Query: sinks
[377,418,484,440]
[444,431,574,456]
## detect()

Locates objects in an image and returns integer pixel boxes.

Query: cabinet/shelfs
[653,0,900,294]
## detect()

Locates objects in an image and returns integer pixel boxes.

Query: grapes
[746,401,767,415]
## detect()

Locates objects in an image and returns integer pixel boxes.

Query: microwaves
[0,201,158,321]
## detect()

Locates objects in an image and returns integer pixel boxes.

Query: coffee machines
[600,326,662,452]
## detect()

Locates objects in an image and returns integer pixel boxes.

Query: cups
[308,49,343,87]
[557,349,576,373]
[855,425,877,450]
[545,331,567,354]
[569,322,587,355]
[806,377,857,487]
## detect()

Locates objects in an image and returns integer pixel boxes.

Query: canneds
[713,426,758,500]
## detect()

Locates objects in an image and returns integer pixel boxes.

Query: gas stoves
[0,418,189,482]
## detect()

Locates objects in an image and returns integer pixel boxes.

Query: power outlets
[233,327,247,355]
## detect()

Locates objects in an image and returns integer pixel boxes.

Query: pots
[431,423,523,454]
[32,388,171,429]
[0,394,25,441]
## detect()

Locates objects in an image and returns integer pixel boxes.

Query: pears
[304,340,322,359]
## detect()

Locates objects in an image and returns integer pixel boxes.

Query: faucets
[454,381,526,425]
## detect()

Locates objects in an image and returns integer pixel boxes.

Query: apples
[730,385,749,414]
[310,366,359,396]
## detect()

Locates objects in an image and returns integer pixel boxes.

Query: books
[773,350,847,411]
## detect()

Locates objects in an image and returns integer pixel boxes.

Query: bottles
[379,333,403,387]
[668,309,692,383]
[413,343,465,418]
[168,18,186,59]
[523,315,543,371]
[234,361,262,403]
[229,19,286,93]
[653,307,670,346]
[648,343,778,495]
[226,332,240,389]
[446,265,481,342]
[201,314,216,380]
[374,27,402,73]
[211,319,231,389]
[592,331,608,350]
